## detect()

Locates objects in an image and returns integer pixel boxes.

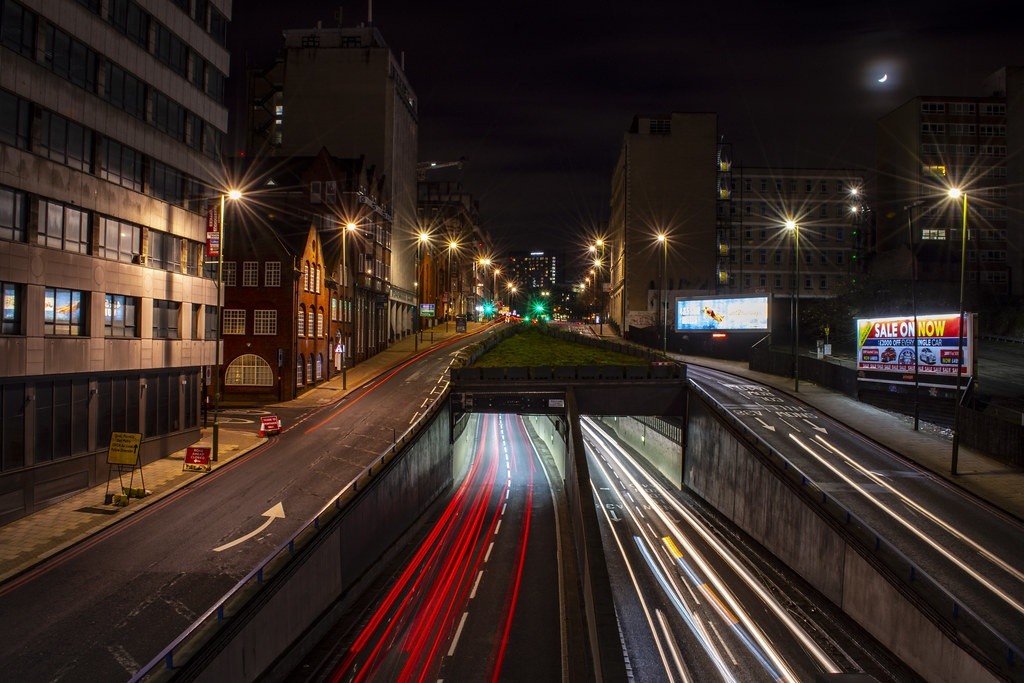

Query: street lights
[657,234,668,355]
[948,189,968,475]
[213,189,241,462]
[448,240,518,307]
[579,236,606,289]
[341,223,356,390]
[415,233,428,353]
[785,222,800,392]
